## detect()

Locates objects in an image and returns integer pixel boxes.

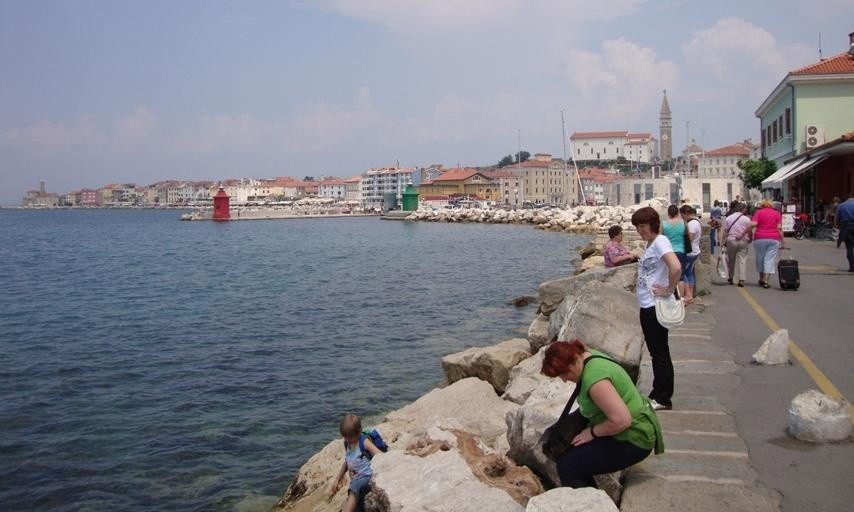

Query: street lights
[700,127,706,179]
[686,120,692,174]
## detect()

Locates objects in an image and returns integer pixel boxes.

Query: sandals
[650,400,672,410]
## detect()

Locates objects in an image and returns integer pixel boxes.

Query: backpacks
[344,429,388,458]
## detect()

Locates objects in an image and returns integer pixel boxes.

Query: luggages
[778,248,800,290]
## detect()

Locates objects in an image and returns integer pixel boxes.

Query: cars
[691,201,729,218]
[442,200,605,211]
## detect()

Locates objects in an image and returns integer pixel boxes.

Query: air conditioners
[806,124,824,136]
[806,137,824,148]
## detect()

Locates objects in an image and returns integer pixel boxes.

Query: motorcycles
[793,199,825,240]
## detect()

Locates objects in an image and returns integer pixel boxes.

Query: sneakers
[760,281,770,288]
[728,279,733,284]
[739,281,744,287]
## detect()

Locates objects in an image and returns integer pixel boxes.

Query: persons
[731,195,740,210]
[711,200,722,246]
[604,225,640,267]
[815,194,854,272]
[749,198,784,288]
[332,415,389,512]
[632,207,682,410]
[680,204,702,303]
[724,203,752,287]
[541,341,664,490]
[660,205,688,297]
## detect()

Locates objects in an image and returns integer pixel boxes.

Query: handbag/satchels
[685,233,692,252]
[717,248,729,279]
[655,300,685,329]
[542,410,589,458]
